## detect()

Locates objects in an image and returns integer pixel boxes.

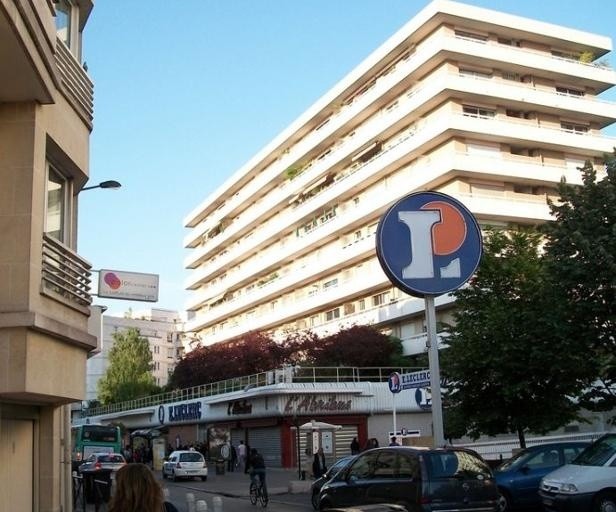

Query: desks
[81,180,122,191]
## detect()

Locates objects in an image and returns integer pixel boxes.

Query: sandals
[71,424,123,476]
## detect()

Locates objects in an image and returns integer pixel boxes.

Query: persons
[351,437,360,454]
[123,440,209,464]
[244,440,250,455]
[389,436,400,445]
[311,447,327,478]
[244,448,271,501]
[105,461,179,511]
[209,426,230,448]
[236,440,247,472]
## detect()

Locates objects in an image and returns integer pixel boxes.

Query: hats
[311,444,506,512]
[162,450,208,482]
[77,452,128,479]
[493,433,616,512]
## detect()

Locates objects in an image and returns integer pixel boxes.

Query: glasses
[73,181,122,199]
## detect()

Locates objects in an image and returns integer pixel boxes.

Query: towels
[216,460,225,475]
[84,467,113,504]
